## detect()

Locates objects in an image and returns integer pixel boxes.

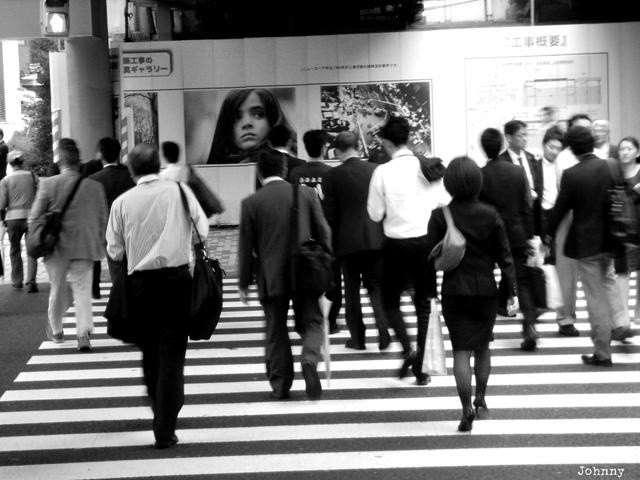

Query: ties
[518,158,534,207]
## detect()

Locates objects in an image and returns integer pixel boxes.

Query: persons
[255,126,307,188]
[321,131,390,352]
[366,114,452,387]
[426,155,520,434]
[0,128,9,181]
[206,87,297,164]
[158,139,192,185]
[27,133,136,352]
[287,128,343,335]
[237,150,333,400]
[0,150,41,294]
[480,113,640,369]
[104,143,207,448]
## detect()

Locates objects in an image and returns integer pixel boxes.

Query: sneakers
[610,326,634,340]
[399,349,431,385]
[343,335,392,351]
[498,306,516,318]
[46,326,64,342]
[300,360,321,398]
[582,354,613,367]
[270,387,290,400]
[28,284,39,291]
[521,337,537,351]
[77,337,92,351]
[560,323,580,336]
[13,282,23,288]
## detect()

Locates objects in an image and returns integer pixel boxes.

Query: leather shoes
[155,434,178,448]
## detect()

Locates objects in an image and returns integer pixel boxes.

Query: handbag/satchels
[521,264,563,309]
[606,187,640,275]
[25,210,62,259]
[293,237,336,298]
[188,173,224,218]
[429,225,467,272]
[189,258,223,340]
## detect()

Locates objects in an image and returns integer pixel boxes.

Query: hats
[7,150,24,164]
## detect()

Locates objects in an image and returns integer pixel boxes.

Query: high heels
[472,397,488,411]
[459,410,475,432]
